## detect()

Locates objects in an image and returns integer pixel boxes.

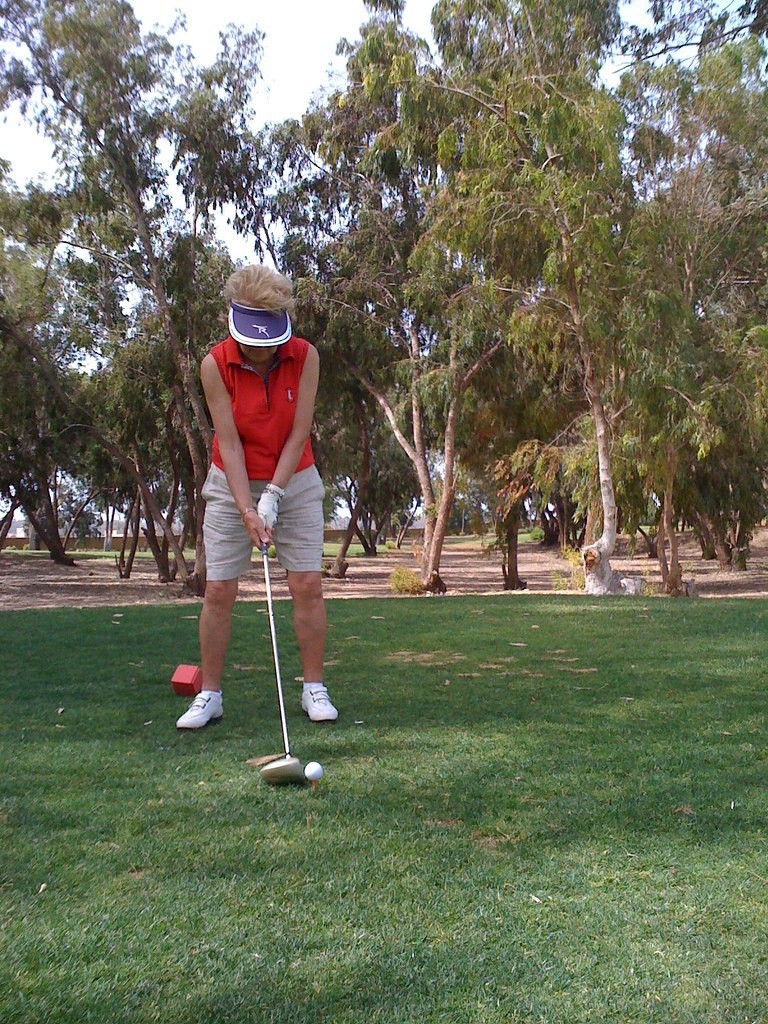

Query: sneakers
[176,690,224,729]
[301,686,338,720]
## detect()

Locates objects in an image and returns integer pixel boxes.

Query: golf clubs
[259,540,305,785]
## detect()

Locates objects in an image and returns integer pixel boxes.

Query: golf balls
[304,762,324,782]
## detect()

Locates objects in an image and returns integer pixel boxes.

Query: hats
[226,297,292,347]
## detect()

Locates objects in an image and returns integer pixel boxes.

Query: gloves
[255,483,285,531]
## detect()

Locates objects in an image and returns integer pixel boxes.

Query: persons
[176,264,338,730]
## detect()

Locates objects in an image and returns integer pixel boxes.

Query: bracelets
[239,508,258,528]
